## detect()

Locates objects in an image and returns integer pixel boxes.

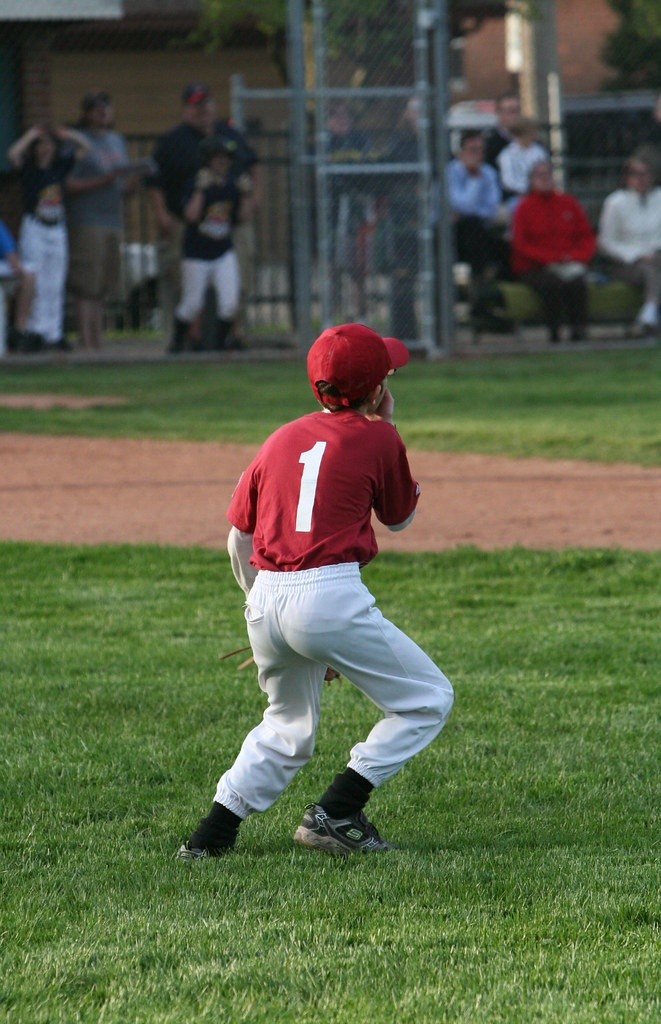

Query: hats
[182,82,210,109]
[308,323,408,408]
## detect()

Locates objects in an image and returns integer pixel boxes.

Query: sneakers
[176,841,208,862]
[293,801,395,856]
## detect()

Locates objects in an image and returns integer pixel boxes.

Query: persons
[0,91,661,348]
[176,322,455,859]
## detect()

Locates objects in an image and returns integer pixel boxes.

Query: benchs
[488,280,643,341]
